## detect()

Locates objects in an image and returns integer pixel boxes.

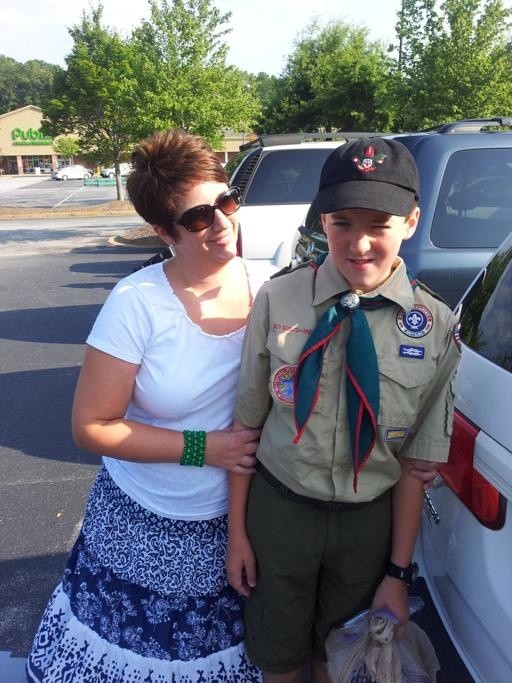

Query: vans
[412,229,512,683]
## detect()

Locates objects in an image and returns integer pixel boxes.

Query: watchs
[383,561,419,589]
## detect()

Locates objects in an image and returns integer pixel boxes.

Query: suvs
[221,131,433,304]
[291,117,512,312]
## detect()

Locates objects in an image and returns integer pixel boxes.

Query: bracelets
[179,430,207,468]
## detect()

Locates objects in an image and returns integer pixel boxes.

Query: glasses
[170,186,244,234]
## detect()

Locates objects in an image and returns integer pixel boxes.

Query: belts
[255,462,398,515]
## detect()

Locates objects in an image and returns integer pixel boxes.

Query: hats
[312,134,417,217]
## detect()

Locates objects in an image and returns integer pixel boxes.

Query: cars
[51,164,92,181]
[100,162,131,178]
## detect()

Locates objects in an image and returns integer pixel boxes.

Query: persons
[227,133,464,682]
[23,125,284,683]
